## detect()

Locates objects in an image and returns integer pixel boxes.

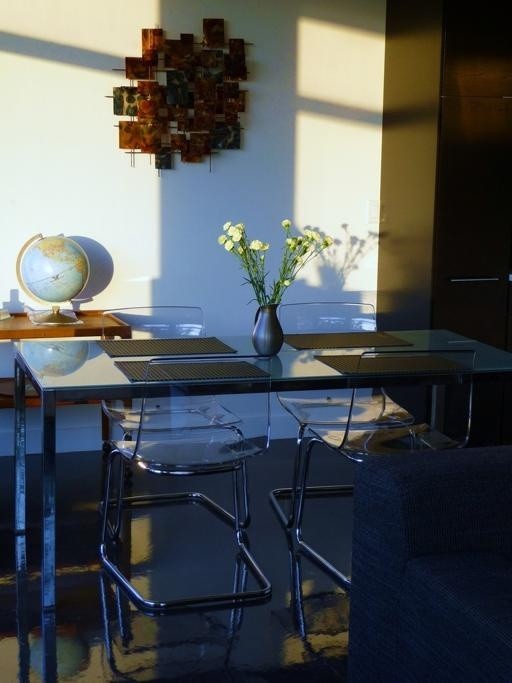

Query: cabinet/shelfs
[1,308,140,473]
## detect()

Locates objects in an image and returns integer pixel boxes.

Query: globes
[20,340,88,377]
[15,232,92,324]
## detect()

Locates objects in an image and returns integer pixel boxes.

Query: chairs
[267,298,416,534]
[96,352,282,616]
[293,345,478,589]
[336,444,512,682]
[93,301,254,538]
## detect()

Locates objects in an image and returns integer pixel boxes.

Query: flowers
[217,219,334,305]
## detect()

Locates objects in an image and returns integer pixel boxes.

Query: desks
[9,329,512,607]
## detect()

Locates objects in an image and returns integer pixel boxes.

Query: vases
[251,303,284,358]
